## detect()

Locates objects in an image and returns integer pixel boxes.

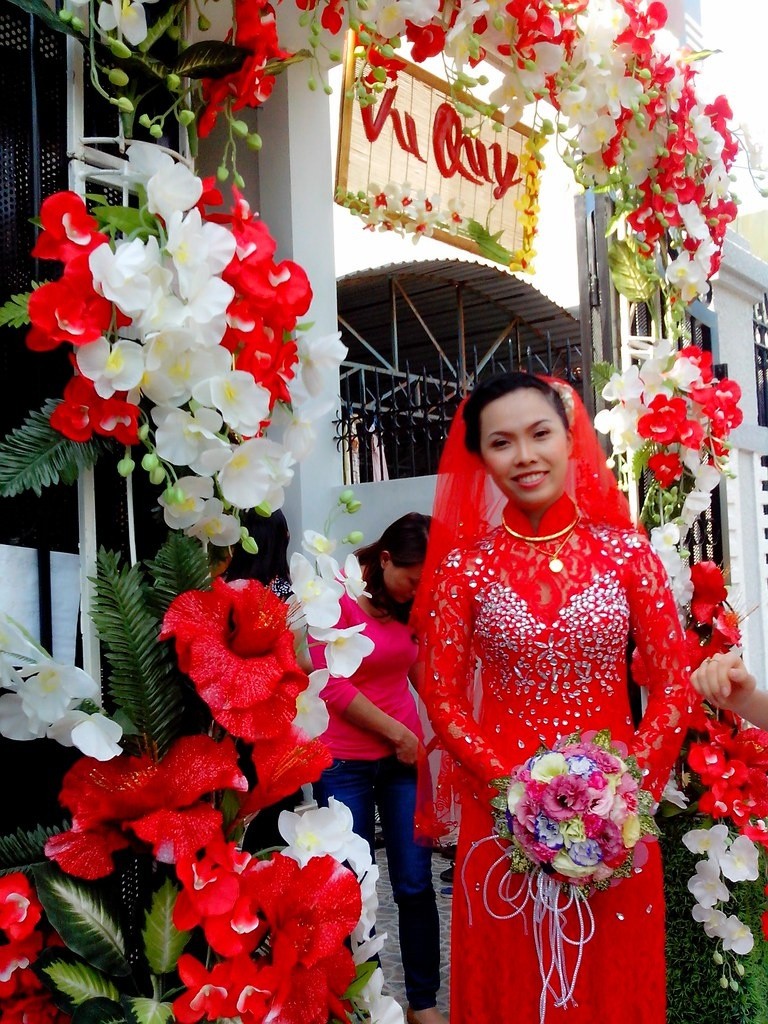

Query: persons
[689,652,768,730]
[308,512,445,1024]
[409,374,692,1024]
[216,508,303,862]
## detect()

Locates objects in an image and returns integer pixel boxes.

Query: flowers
[486,725,663,905]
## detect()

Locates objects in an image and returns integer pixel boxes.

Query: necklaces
[500,514,577,541]
[524,516,581,572]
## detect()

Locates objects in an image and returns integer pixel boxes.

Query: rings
[706,657,719,663]
